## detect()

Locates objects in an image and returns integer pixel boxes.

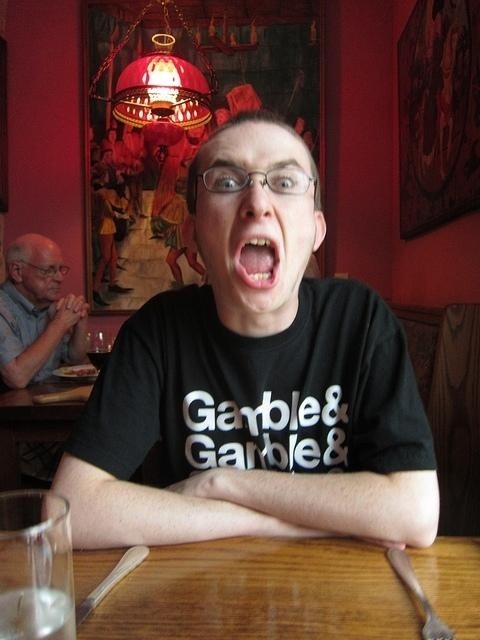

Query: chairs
[383,294,480,535]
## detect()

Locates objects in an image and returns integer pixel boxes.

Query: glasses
[197,165,315,193]
[20,259,70,278]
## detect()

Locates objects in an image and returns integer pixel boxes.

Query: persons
[1,233,91,526]
[40,109,439,552]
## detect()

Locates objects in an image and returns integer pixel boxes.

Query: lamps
[87,0,220,162]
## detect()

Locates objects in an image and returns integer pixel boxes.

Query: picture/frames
[397,0,480,240]
[78,0,326,319]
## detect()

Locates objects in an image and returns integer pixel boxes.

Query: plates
[51,364,101,381]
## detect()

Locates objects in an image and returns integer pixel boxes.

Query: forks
[387,546,454,640]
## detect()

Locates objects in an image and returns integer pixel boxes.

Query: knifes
[75,546,151,626]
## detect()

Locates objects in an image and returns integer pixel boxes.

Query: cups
[87,330,114,372]
[0,490,75,640]
